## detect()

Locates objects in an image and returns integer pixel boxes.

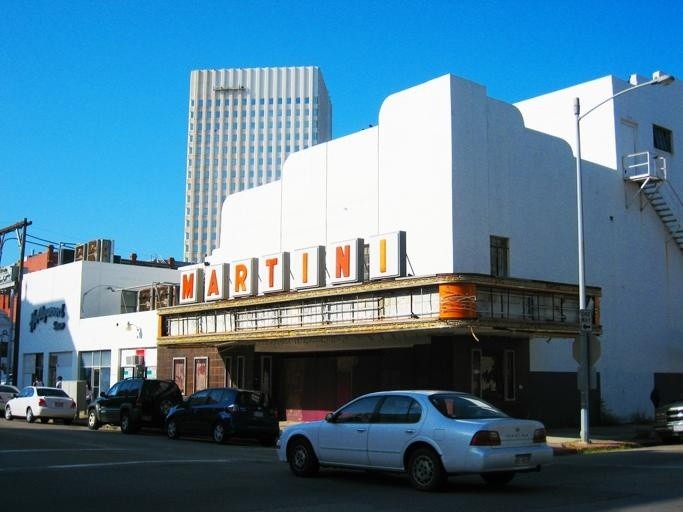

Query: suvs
[85,373,184,435]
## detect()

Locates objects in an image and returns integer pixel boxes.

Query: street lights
[570,71,679,447]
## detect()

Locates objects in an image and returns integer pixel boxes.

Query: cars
[274,389,554,489]
[4,386,76,423]
[654,397,683,442]
[165,386,280,447]
[0,384,23,416]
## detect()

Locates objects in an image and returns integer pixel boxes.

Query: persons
[32,377,44,386]
[85,381,92,404]
[56,376,62,388]
